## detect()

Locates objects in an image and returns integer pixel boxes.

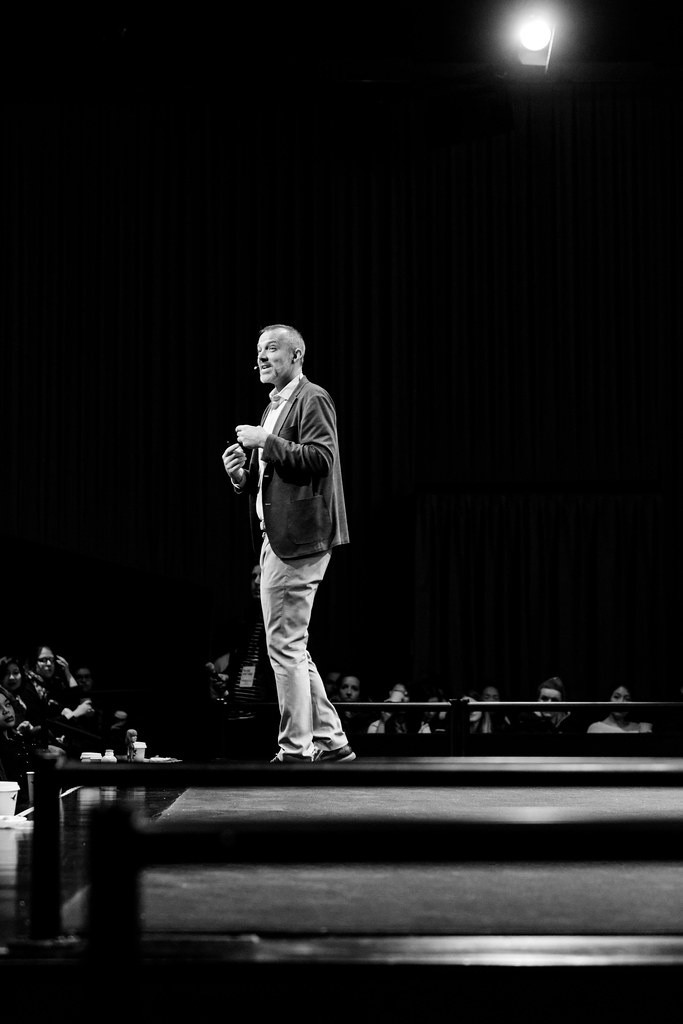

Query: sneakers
[313,744,356,763]
[270,752,313,764]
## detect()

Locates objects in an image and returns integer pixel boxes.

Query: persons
[26,644,94,762]
[329,674,374,734]
[510,677,577,732]
[365,681,431,734]
[415,690,445,734]
[0,657,54,757]
[124,729,137,761]
[323,671,340,697]
[468,685,509,733]
[587,681,652,733]
[75,666,94,691]
[221,324,356,763]
[0,686,39,817]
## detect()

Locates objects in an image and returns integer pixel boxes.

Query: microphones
[205,661,228,692]
[254,366,260,370]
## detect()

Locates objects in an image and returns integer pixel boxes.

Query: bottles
[101,749,117,790]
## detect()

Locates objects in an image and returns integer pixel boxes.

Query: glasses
[35,657,56,662]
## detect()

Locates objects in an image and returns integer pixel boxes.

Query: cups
[128,742,147,762]
[90,757,102,763]
[81,751,101,762]
[0,781,20,816]
[638,722,652,733]
[27,772,34,803]
